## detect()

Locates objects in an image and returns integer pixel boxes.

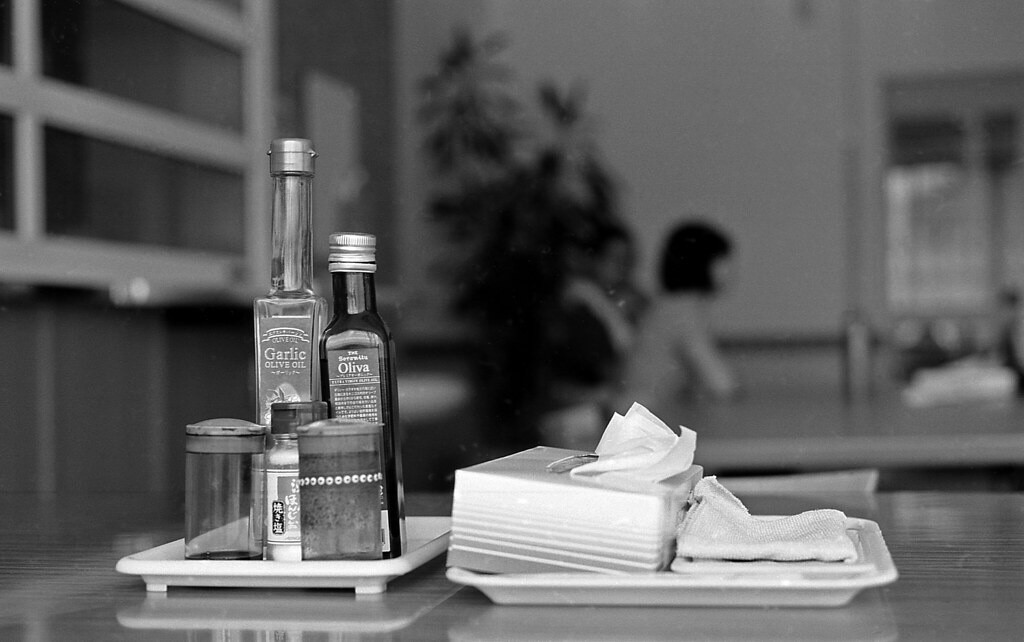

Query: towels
[675,475,858,564]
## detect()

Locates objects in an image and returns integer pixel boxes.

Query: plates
[116,516,450,594]
[445,516,899,604]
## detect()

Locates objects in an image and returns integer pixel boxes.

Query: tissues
[446,401,705,574]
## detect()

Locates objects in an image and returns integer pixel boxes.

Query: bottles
[297,417,386,559]
[186,417,267,560]
[254,139,328,427]
[320,232,406,559]
[252,401,328,545]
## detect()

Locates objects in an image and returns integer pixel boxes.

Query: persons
[529,216,754,452]
